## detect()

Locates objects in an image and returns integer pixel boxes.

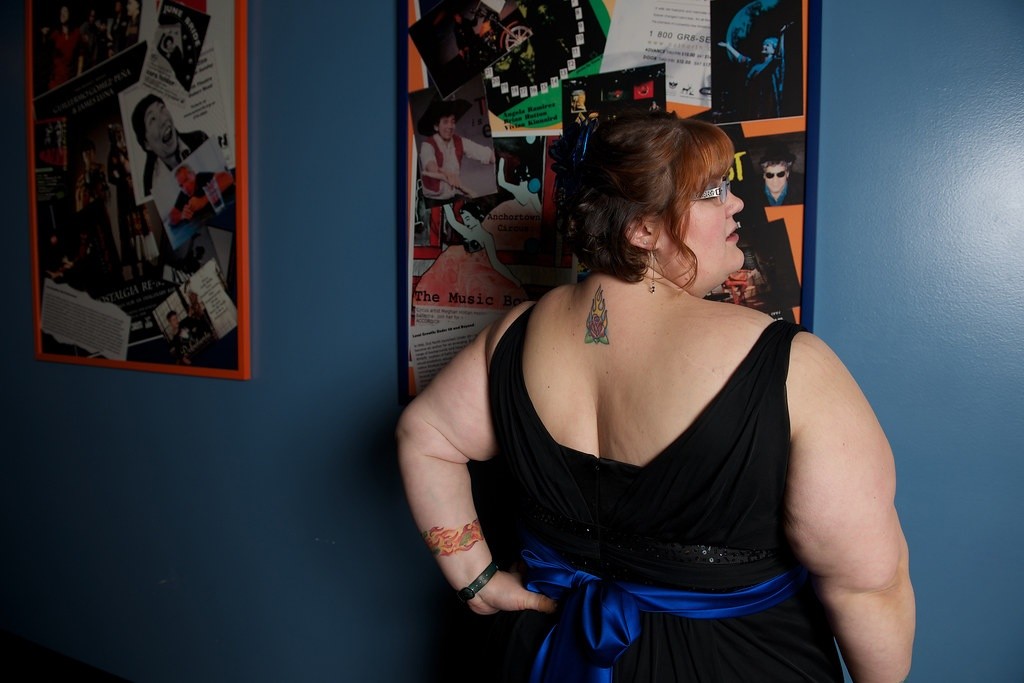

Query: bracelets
[455,561,498,601]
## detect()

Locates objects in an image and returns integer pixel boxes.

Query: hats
[759,143,796,163]
[417,92,473,136]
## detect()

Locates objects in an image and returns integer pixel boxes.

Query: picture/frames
[389,0,822,402]
[22,0,252,382]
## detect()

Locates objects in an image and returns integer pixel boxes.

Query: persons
[166,293,212,364]
[420,112,496,207]
[454,12,496,60]
[158,33,182,66]
[759,149,804,206]
[41,0,142,90]
[168,165,233,226]
[75,123,147,295]
[718,23,794,108]
[395,112,917,683]
[131,93,208,195]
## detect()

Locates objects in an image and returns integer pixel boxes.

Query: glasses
[691,177,731,204]
[766,172,785,178]
